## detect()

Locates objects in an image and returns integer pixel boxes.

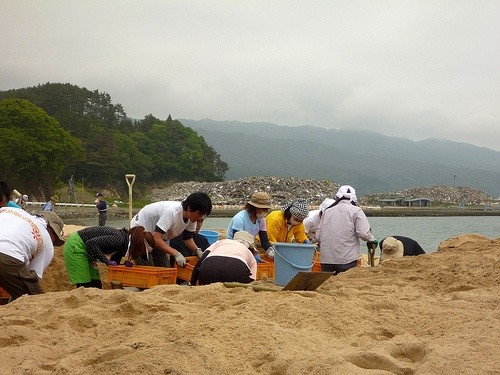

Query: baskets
[174,256,200,286]
[109,266,177,288]
[255,254,275,280]
[312,252,322,272]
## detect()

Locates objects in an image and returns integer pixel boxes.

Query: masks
[255,210,267,219]
[290,216,300,226]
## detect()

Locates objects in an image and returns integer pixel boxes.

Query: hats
[51,195,60,202]
[382,237,403,261]
[94,193,103,197]
[40,212,65,247]
[319,198,336,213]
[248,191,273,209]
[234,230,259,254]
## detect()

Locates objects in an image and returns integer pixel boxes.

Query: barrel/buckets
[199,230,220,245]
[271,243,318,287]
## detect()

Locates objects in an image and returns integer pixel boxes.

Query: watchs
[195,248,202,255]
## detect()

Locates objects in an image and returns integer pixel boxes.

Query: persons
[190,230,259,286]
[0,180,425,307]
[316,185,377,275]
[94,192,107,226]
[62,226,155,289]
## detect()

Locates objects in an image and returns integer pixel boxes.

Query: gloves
[105,259,117,266]
[175,252,186,266]
[124,260,134,267]
[367,240,378,249]
[304,239,309,244]
[196,248,203,258]
[254,254,262,264]
